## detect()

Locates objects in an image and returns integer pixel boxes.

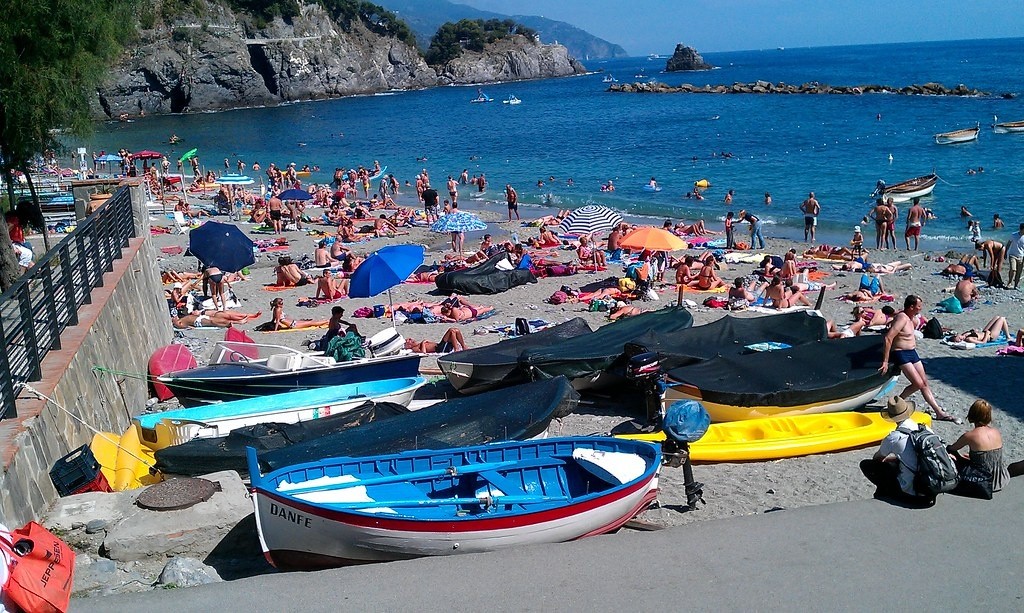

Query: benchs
[477,470,541,509]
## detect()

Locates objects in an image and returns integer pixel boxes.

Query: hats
[880,396,916,423]
[290,163,297,166]
[414,174,422,179]
[853,225,862,233]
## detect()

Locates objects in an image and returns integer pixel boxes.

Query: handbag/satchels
[0,520,76,613]
[945,458,993,500]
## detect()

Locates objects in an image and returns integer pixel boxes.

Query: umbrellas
[277,188,314,200]
[429,212,487,232]
[129,149,163,159]
[617,226,689,251]
[94,154,124,162]
[215,172,256,184]
[558,206,623,272]
[190,221,255,273]
[348,245,425,327]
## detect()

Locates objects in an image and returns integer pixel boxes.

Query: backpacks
[893,423,961,498]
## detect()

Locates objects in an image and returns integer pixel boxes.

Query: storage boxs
[71,470,108,493]
[47,443,102,498]
[105,483,114,492]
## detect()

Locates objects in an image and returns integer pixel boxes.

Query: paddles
[281,459,520,496]
[324,495,569,509]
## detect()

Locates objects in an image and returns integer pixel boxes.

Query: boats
[471,98,494,104]
[614,410,934,465]
[990,114,1024,135]
[342,165,388,183]
[131,375,428,446]
[503,99,522,105]
[156,346,428,409]
[932,120,981,146]
[245,429,662,574]
[155,312,904,478]
[869,167,940,206]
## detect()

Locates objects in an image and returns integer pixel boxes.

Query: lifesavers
[643,184,663,192]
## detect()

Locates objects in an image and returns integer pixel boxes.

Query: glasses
[861,310,865,313]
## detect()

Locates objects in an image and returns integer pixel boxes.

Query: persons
[1016,328,1024,347]
[6,109,1003,354]
[955,272,978,306]
[943,253,979,274]
[1004,224,1024,289]
[871,395,932,500]
[947,401,1010,492]
[949,316,1013,344]
[877,295,955,422]
[976,241,1005,277]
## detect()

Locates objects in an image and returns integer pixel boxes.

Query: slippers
[939,414,953,421]
